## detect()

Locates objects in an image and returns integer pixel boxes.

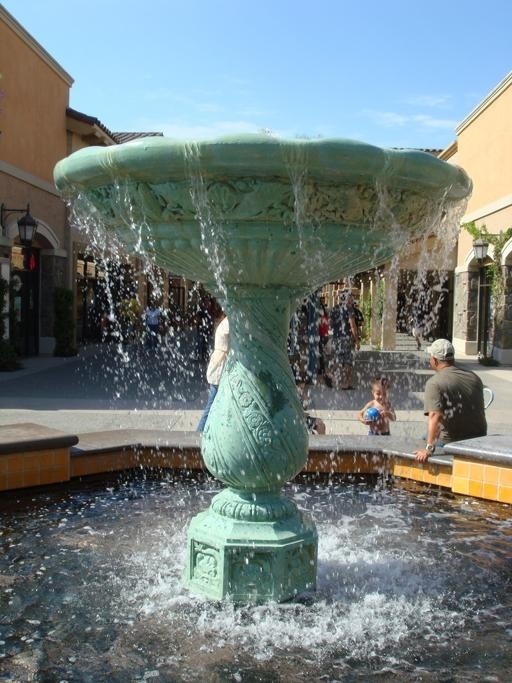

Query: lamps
[472,232,512,265]
[0,201,39,246]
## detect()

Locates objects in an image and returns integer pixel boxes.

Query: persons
[99,282,365,390]
[411,338,487,464]
[408,311,424,350]
[196,313,230,433]
[293,377,326,436]
[358,381,396,436]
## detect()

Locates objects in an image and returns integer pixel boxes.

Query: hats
[424,338,455,359]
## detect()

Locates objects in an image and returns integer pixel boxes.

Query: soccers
[362,407,380,421]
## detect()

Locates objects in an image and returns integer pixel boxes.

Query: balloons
[362,407,380,424]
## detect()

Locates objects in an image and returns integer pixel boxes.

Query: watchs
[425,443,435,452]
